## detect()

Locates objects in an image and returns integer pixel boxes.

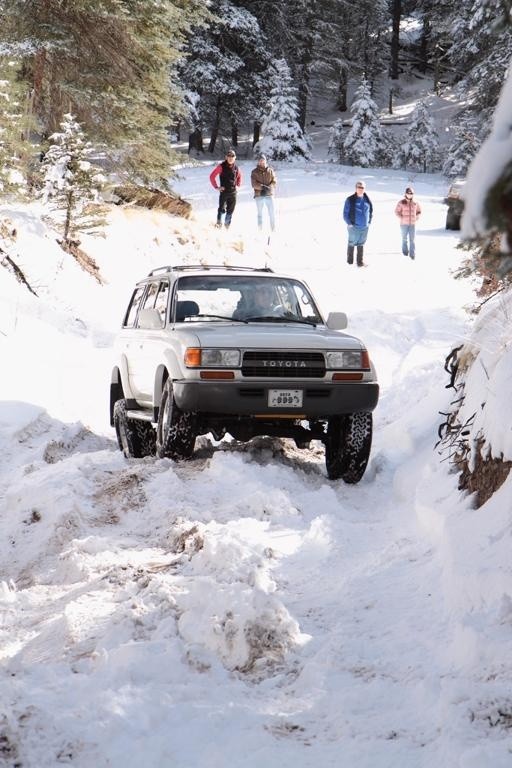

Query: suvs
[108,265,381,485]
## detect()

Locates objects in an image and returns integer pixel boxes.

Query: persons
[232,283,298,321]
[210,150,241,229]
[250,153,276,232]
[441,186,464,231]
[343,181,373,267]
[395,187,421,259]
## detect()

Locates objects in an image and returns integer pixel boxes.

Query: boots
[357,246,364,267]
[347,246,353,264]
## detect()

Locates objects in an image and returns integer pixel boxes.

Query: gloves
[260,187,268,197]
[261,185,270,192]
